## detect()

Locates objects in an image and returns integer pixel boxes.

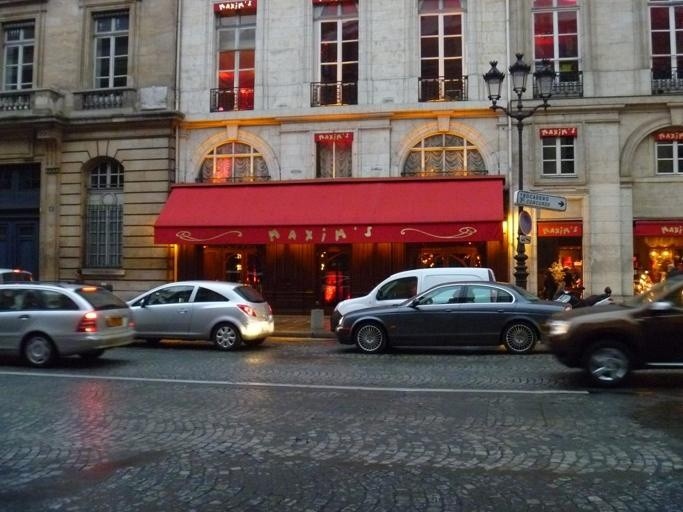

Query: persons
[542,269,554,300]
[73,270,84,283]
[560,267,573,289]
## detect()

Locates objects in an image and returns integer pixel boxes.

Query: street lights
[481,49,558,293]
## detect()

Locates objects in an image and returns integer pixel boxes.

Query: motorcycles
[552,285,615,310]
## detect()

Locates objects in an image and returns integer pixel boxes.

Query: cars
[543,268,682,398]
[0,278,139,373]
[331,279,576,356]
[125,279,277,354]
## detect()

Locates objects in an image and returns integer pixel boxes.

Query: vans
[0,265,33,284]
[326,265,499,333]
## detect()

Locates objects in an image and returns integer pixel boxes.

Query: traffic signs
[512,189,567,213]
